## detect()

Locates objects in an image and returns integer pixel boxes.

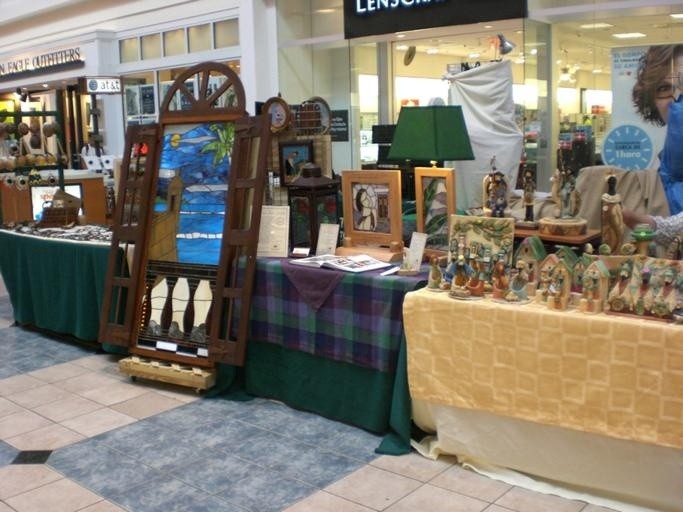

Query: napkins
[280,261,344,310]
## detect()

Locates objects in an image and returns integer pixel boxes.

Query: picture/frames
[339,168,457,261]
[278,141,314,188]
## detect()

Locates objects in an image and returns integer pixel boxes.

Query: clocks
[601,123,653,173]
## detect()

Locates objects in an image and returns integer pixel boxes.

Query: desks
[0,228,448,456]
[515,228,604,255]
[403,255,680,511]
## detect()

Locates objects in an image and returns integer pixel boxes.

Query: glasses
[655,83,683,100]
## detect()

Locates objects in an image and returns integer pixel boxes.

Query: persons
[480,161,583,223]
[630,44,683,127]
[617,202,683,253]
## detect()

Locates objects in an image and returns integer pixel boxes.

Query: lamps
[386,106,475,163]
[16,86,41,102]
[496,31,516,58]
